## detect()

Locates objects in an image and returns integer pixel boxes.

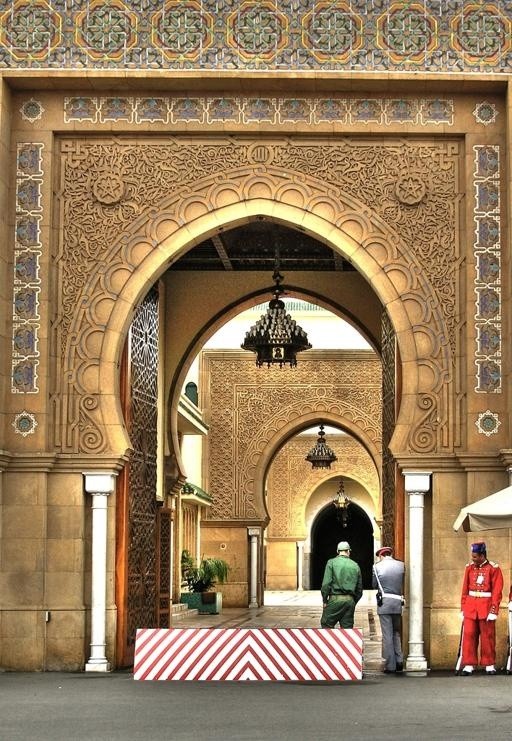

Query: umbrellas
[450,484,512,534]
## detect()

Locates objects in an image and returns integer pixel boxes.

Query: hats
[376,547,393,557]
[471,543,486,552]
[337,541,352,551]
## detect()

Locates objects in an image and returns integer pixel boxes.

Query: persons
[320,541,364,630]
[372,546,405,675]
[458,541,504,672]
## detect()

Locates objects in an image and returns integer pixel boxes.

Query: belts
[329,594,353,600]
[382,593,402,600]
[469,590,492,598]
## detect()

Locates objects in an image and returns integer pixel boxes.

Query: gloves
[486,613,498,621]
[460,611,464,621]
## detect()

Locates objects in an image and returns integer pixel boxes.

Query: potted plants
[180,548,231,614]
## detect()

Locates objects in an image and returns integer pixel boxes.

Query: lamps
[332,479,352,521]
[241,224,312,368]
[305,423,338,470]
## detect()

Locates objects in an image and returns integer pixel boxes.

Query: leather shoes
[383,662,403,673]
[485,665,496,675]
[462,666,476,676]
